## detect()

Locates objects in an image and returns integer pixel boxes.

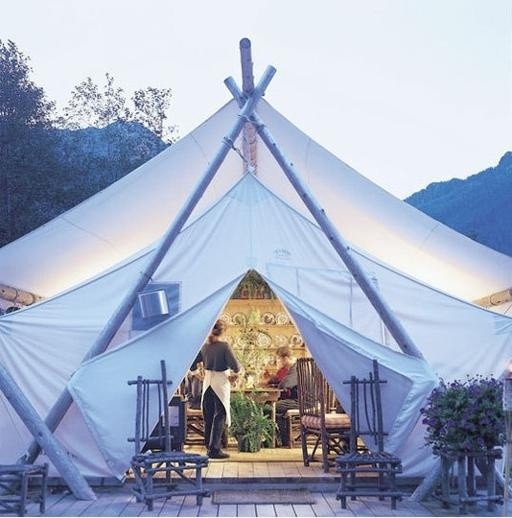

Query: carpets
[213,487,316,504]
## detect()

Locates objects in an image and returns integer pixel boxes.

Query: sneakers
[210,451,229,458]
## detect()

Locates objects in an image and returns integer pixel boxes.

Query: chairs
[335,359,402,511]
[296,357,354,474]
[128,359,210,511]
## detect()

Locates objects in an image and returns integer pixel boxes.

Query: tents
[0,36,512,478]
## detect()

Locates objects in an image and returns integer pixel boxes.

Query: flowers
[421,378,510,458]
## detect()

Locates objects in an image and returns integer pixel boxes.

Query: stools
[286,408,324,448]
[0,462,49,517]
[184,408,206,445]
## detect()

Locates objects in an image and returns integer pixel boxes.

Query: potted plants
[229,395,280,453]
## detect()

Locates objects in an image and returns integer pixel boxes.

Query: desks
[226,390,279,453]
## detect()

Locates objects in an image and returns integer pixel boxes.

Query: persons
[189,319,241,458]
[268,346,316,446]
[256,346,294,399]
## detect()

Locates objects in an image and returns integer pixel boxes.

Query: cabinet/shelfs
[181,299,321,430]
